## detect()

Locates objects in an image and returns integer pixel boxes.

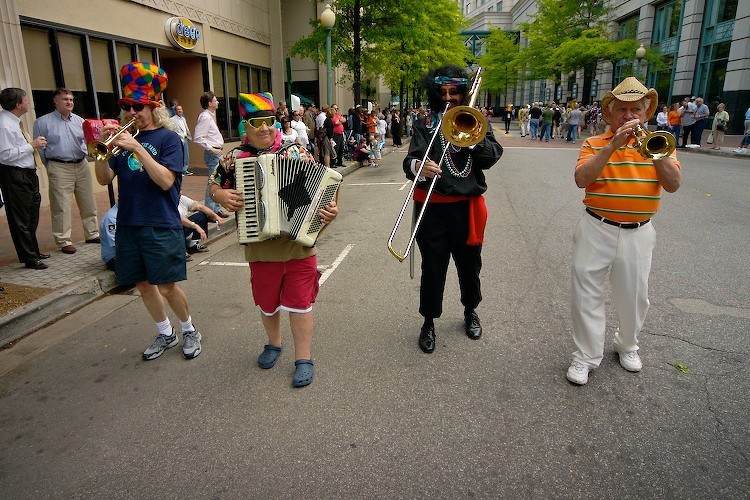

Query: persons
[566,77,681,384]
[733,107,750,153]
[170,105,194,177]
[177,195,225,259]
[0,87,50,269]
[168,98,178,117]
[32,87,101,254]
[99,200,118,273]
[95,61,201,360]
[476,106,494,123]
[402,66,503,354]
[502,96,709,150]
[207,92,338,388]
[277,100,436,168]
[238,119,247,143]
[194,91,230,218]
[711,103,730,149]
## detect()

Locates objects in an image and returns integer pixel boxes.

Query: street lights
[320,3,336,107]
[635,43,647,81]
[568,69,576,99]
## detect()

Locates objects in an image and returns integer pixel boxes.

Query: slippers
[293,359,313,387]
[258,345,282,369]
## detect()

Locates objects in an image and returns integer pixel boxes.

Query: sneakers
[733,147,749,154]
[142,326,179,360]
[566,361,591,385]
[182,324,202,359]
[613,343,642,372]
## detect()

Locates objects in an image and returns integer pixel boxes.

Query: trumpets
[86,116,140,163]
[622,119,677,161]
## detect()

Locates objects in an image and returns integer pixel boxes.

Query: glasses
[120,102,144,111]
[293,114,300,116]
[247,115,276,129]
[334,108,339,110]
[439,90,460,95]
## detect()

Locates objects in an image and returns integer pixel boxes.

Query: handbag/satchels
[717,124,728,132]
[706,133,714,144]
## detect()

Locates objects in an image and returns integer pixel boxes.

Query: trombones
[387,65,484,263]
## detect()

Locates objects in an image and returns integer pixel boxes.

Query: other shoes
[330,162,378,168]
[505,131,576,144]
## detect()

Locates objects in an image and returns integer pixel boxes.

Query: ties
[20,120,48,186]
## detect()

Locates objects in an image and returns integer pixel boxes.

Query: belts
[48,157,85,164]
[585,208,650,229]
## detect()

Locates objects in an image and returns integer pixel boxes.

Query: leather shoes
[463,312,482,340]
[26,237,100,269]
[419,322,436,353]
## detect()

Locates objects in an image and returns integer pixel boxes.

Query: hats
[119,61,169,107]
[238,91,276,119]
[600,77,658,125]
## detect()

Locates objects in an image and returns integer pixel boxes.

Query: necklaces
[439,126,473,178]
